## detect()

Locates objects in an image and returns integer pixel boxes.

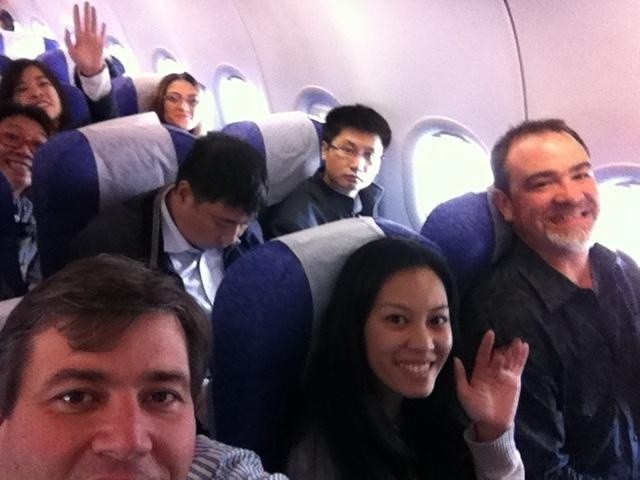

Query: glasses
[0,127,48,158]
[328,143,379,166]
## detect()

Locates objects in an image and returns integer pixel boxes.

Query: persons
[0,6,15,33]
[0,252,293,479]
[271,235,531,480]
[1,103,56,304]
[268,103,393,239]
[0,0,120,136]
[62,129,270,432]
[471,120,639,479]
[152,71,205,136]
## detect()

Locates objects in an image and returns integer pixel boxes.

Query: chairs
[219,110,327,208]
[210,215,440,475]
[421,192,520,267]
